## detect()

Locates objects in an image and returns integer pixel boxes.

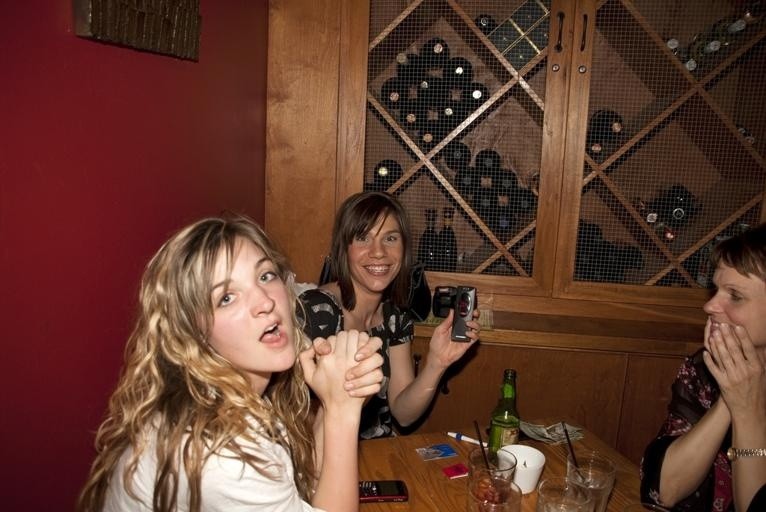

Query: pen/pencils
[443,431,488,448]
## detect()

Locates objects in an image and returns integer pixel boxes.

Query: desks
[357,417,655,512]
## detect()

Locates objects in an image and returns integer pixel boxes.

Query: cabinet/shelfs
[263,0,766,467]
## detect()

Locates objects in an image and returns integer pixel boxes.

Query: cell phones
[358,479,409,502]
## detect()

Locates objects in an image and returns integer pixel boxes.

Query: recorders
[432,286,477,319]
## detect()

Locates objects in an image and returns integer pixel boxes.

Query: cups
[467,445,524,512]
[495,443,548,495]
[535,448,616,511]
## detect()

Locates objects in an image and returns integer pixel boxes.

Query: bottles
[457,2,551,76]
[416,209,439,272]
[488,368,523,468]
[376,37,492,148]
[586,107,624,155]
[571,181,696,286]
[442,141,540,243]
[439,206,458,272]
[375,157,402,189]
[658,7,760,76]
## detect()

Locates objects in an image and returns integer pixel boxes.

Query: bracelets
[725,446,766,460]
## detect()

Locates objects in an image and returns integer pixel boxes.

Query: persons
[263,190,483,442]
[72,206,388,511]
[638,220,766,511]
